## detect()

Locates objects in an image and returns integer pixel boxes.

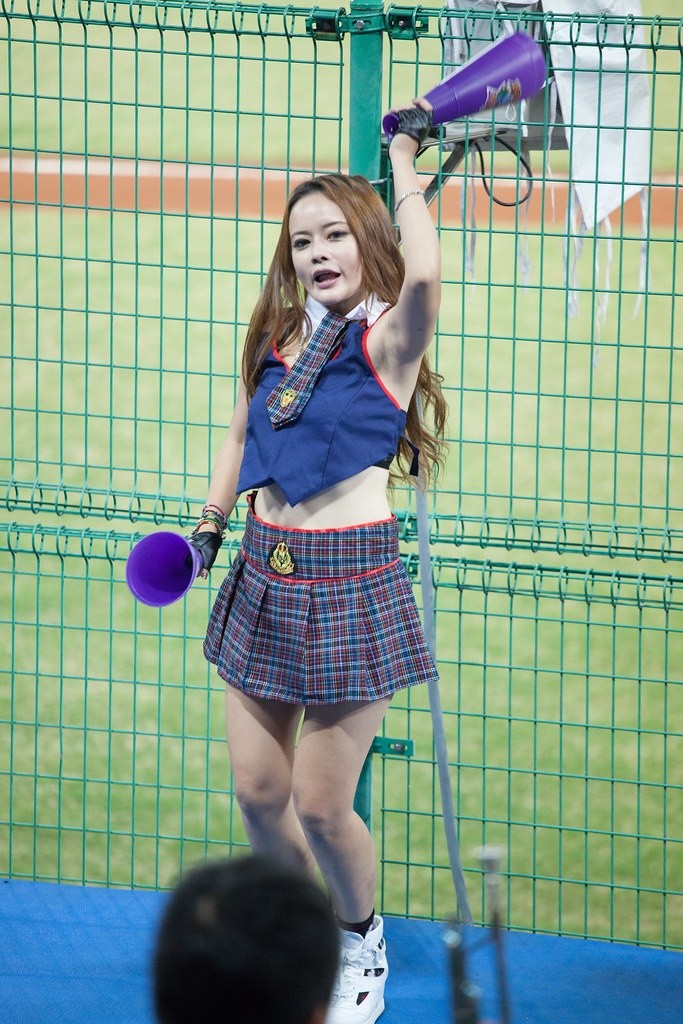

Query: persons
[186,97,442,1024]
[151,848,342,1024]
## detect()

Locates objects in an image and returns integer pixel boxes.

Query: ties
[265,310,351,430]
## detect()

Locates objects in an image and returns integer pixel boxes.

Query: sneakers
[323,915,389,1024]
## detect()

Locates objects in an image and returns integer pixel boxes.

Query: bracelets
[395,190,425,211]
[190,504,228,535]
